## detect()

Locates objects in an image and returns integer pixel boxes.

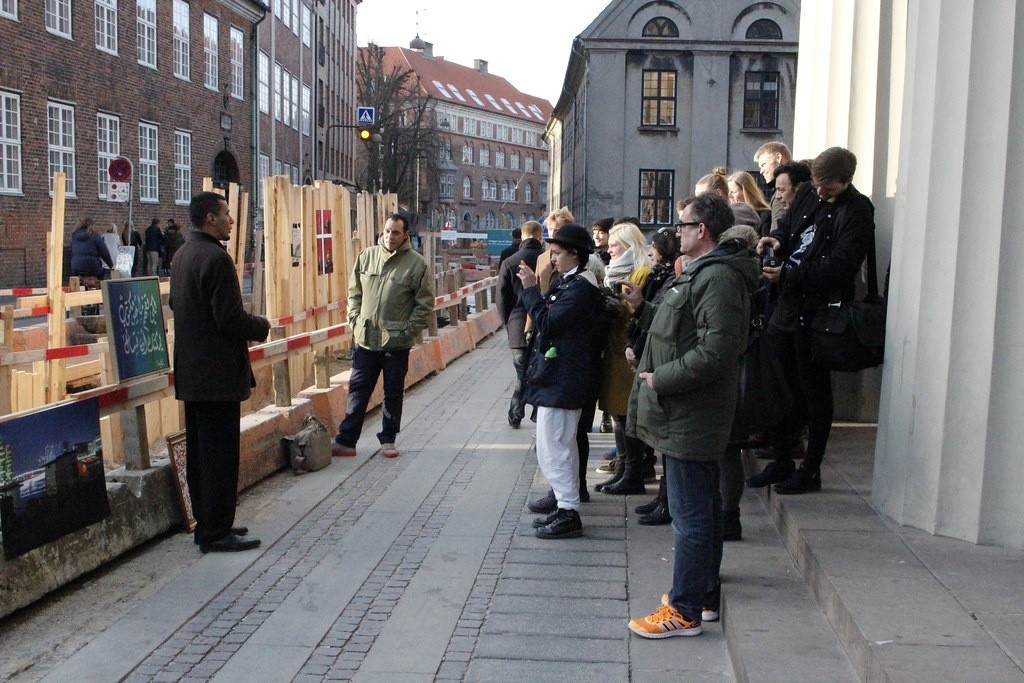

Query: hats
[166,219,174,223]
[592,217,614,234]
[729,202,762,228]
[544,224,595,254]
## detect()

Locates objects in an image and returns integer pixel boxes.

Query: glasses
[674,222,700,232]
[658,227,668,236]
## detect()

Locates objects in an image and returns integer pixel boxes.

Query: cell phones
[613,283,622,294]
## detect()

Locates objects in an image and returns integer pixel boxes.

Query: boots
[634,476,673,525]
[593,450,646,494]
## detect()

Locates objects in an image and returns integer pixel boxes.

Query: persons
[496,140,815,540]
[746,147,874,495]
[168,193,270,553]
[625,192,761,640]
[332,214,435,457]
[515,225,600,539]
[70,216,186,282]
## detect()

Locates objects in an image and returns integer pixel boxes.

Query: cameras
[763,246,780,267]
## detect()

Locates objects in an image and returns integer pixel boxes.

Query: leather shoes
[195,526,248,545]
[533,507,583,538]
[200,532,262,552]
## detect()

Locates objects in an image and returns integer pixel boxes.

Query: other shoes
[508,401,521,429]
[527,493,557,514]
[594,459,615,474]
[771,460,821,494]
[639,456,657,482]
[720,507,742,542]
[603,447,617,459]
[752,438,805,460]
[746,460,795,488]
[600,412,613,433]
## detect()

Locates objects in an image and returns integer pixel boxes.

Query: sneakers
[627,605,703,639]
[331,443,357,457]
[661,594,719,622]
[382,442,399,457]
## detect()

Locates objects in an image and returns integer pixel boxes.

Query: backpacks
[584,268,632,355]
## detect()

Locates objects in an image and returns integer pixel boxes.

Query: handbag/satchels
[727,317,795,445]
[279,414,332,476]
[809,293,887,373]
